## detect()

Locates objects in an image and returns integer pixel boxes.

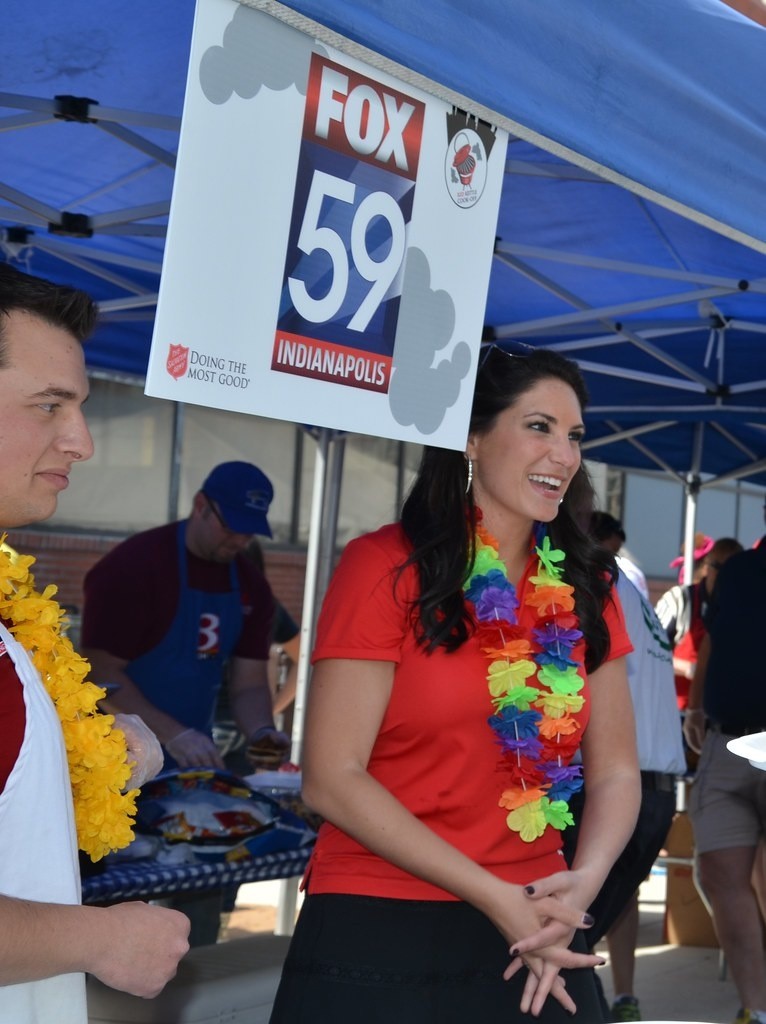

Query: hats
[202,462,276,540]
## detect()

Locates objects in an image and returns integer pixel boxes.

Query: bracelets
[686,708,703,714]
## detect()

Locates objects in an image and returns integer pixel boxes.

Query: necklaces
[461,505,586,842]
[0,531,141,862]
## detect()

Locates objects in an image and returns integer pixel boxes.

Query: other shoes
[611,993,642,1024]
[731,1006,766,1024]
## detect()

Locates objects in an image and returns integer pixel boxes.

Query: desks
[81,797,323,909]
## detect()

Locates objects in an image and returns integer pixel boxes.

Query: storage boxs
[665,812,720,948]
[86,935,295,1024]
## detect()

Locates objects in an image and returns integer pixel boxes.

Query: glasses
[478,339,584,374]
[708,559,721,570]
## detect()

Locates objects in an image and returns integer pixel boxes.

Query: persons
[82,460,301,770]
[0,262,191,1024]
[683,549,766,1024]
[653,537,744,783]
[266,338,642,1024]
[561,508,686,1024]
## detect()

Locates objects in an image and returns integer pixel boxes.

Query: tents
[0,0,766,936]
